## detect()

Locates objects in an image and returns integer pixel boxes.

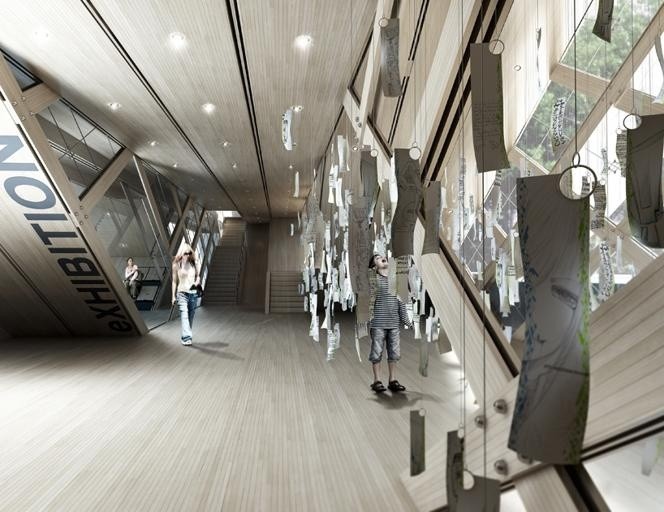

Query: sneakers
[370,380,386,392]
[388,381,405,391]
[180,336,192,345]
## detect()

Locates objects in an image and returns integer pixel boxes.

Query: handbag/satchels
[197,285,205,297]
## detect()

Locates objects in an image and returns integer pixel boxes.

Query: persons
[123,257,139,299]
[366,254,414,392]
[171,243,202,346]
